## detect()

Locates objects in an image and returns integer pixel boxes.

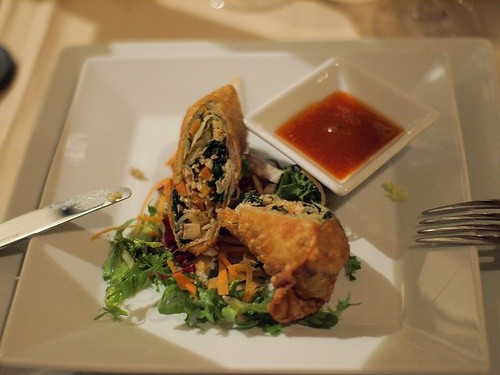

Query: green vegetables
[92,164,363,335]
